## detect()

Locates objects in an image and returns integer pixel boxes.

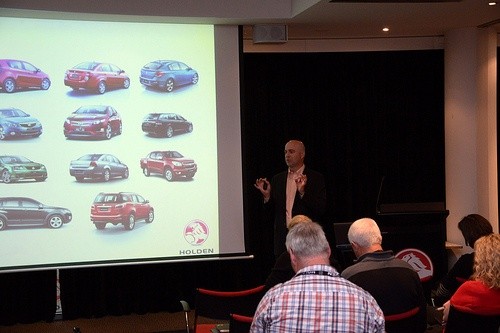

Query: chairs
[384,307,420,333]
[180,285,265,333]
[228,313,254,333]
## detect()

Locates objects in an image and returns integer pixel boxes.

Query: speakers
[252,23,288,43]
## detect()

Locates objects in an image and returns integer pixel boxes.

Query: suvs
[89,191,155,231]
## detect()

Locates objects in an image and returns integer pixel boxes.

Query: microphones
[376,176,386,214]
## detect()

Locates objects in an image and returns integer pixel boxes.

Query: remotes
[263,178,267,190]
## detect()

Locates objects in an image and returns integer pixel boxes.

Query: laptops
[334,222,353,248]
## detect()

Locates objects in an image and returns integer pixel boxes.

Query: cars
[139,60,199,93]
[63,104,123,141]
[140,149,198,182]
[141,112,194,138]
[0,58,51,93]
[0,155,49,184]
[0,105,43,141]
[62,61,131,95]
[69,153,130,182]
[0,196,72,231]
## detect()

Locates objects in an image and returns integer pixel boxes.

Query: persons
[450,214,500,315]
[255,141,335,288]
[250,215,386,333]
[341,218,420,316]
